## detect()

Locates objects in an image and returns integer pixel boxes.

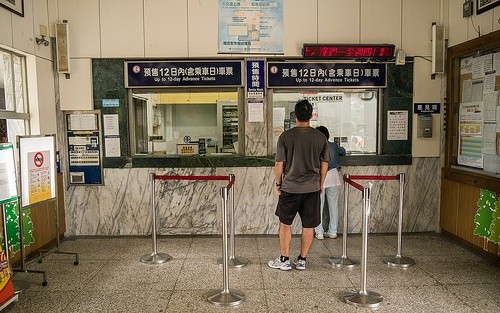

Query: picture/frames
[0,0,25,17]
[476,0,500,15]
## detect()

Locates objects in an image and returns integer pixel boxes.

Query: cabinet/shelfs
[216,100,239,152]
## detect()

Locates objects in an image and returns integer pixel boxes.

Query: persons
[316,125,344,240]
[268,100,328,270]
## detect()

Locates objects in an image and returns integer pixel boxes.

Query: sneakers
[268,255,297,270]
[292,257,306,270]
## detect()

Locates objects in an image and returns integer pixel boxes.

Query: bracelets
[276,182,282,186]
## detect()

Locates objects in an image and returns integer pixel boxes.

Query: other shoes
[325,231,338,238]
[315,234,325,240]
